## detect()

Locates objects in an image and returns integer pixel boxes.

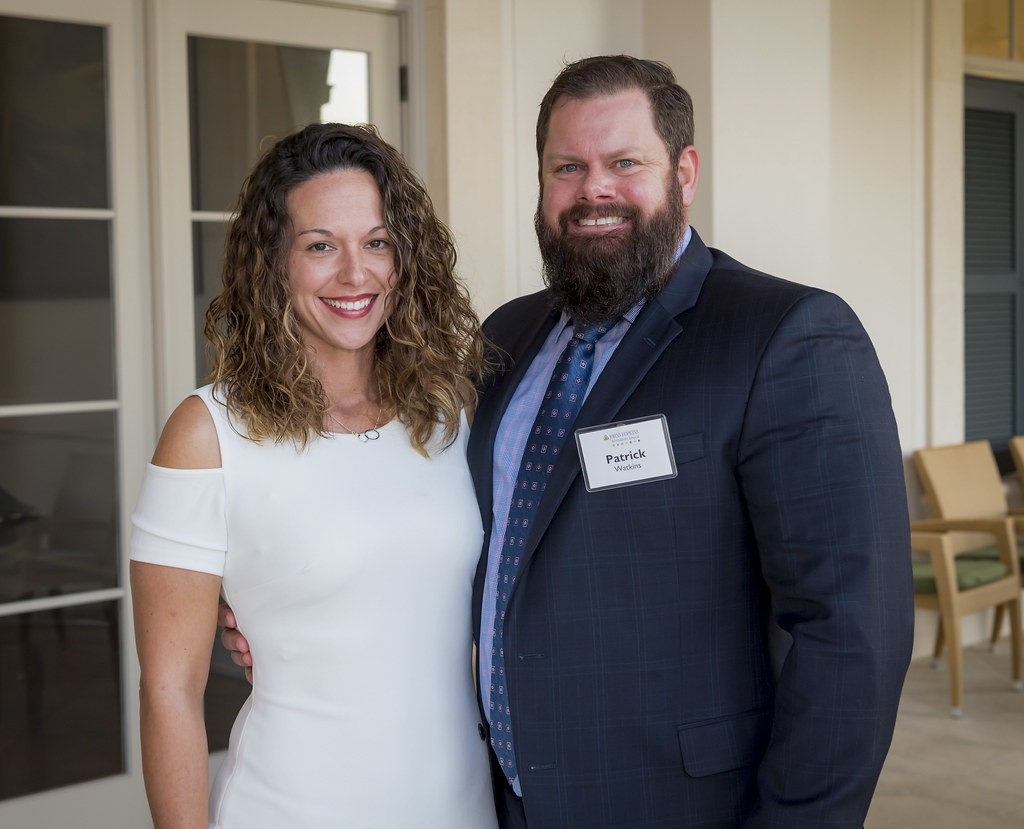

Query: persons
[129,120,492,828]
[215,53,919,829]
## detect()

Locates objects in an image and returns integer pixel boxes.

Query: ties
[489,306,619,786]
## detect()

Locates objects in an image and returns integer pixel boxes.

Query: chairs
[909,435,1024,719]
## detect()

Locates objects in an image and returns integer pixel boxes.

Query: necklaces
[324,384,392,442]
